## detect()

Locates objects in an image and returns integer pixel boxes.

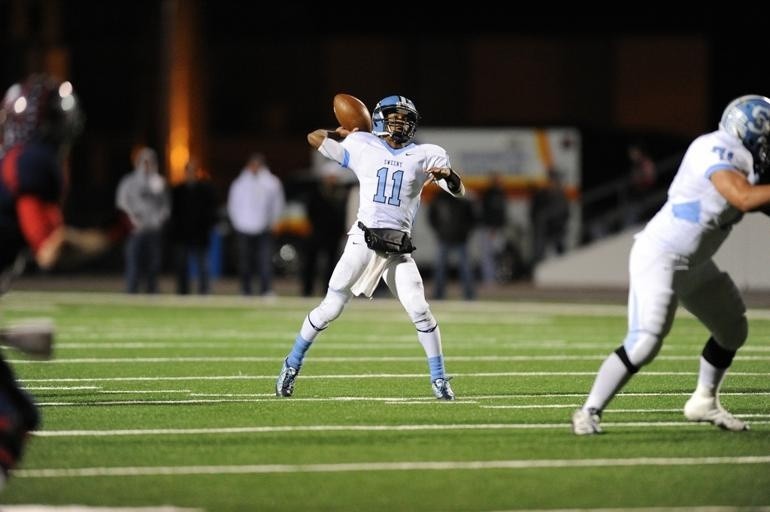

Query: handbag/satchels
[358,222,416,253]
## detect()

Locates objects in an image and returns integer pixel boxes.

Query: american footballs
[333,93,372,132]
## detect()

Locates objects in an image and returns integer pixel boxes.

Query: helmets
[372,95,418,143]
[718,93,770,150]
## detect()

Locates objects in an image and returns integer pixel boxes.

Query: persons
[1,50,134,296]
[0,346,41,472]
[274,96,467,402]
[115,145,360,298]
[572,94,770,435]
[426,146,656,300]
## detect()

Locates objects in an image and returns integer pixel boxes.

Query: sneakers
[432,378,455,400]
[276,357,300,397]
[684,396,750,432]
[570,408,603,436]
